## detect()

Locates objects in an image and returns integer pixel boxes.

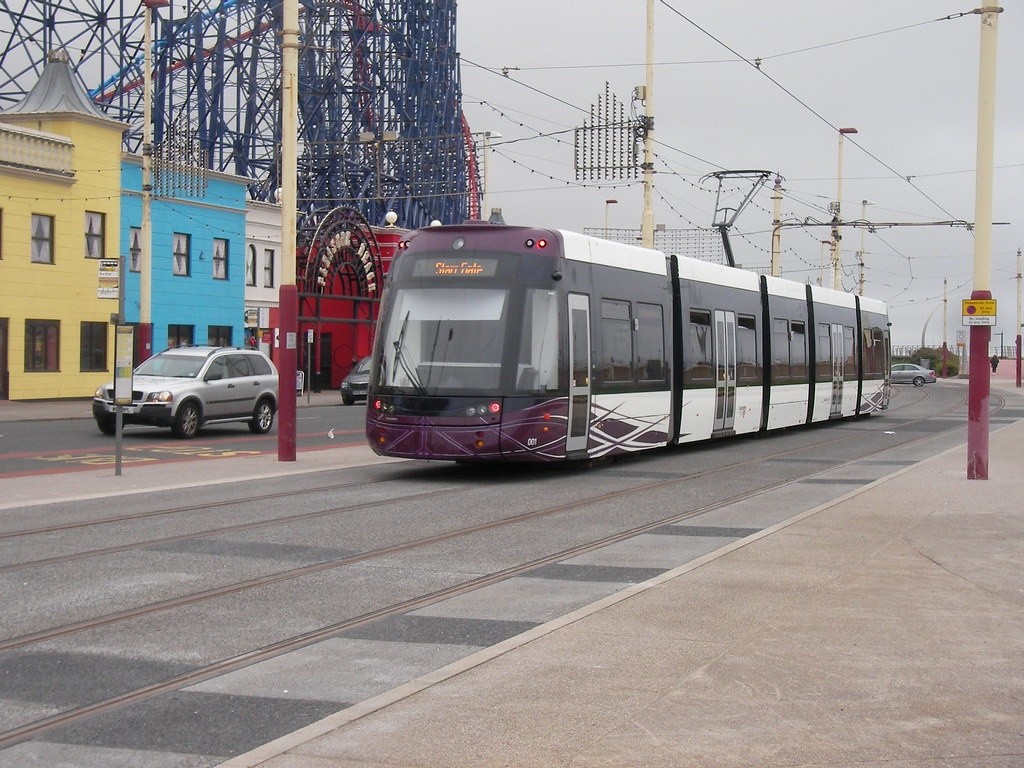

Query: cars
[341,355,372,404]
[892,362,937,387]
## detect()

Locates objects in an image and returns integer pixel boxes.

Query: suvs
[93,344,280,441]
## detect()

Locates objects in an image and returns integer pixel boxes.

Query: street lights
[136,0,174,364]
[604,198,618,240]
[832,127,859,291]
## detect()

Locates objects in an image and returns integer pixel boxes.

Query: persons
[990,355,999,373]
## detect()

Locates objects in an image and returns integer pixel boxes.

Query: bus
[364,205,895,473]
